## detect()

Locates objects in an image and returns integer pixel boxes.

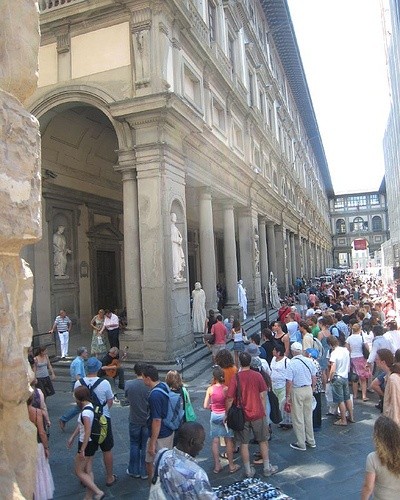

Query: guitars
[105,353,127,377]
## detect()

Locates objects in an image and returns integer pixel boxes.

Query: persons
[72,356,118,488]
[284,341,317,452]
[48,308,72,359]
[30,346,57,400]
[25,268,400,500]
[64,385,105,500]
[358,415,400,500]
[223,352,279,478]
[147,420,222,500]
[99,307,120,350]
[202,368,241,474]
[326,335,356,427]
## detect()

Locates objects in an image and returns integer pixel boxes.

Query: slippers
[230,464,240,473]
[105,474,119,486]
[213,465,224,473]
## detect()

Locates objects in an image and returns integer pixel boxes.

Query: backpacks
[147,382,185,430]
[81,400,108,444]
[79,377,107,416]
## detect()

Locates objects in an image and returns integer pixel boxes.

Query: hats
[245,343,262,356]
[290,342,302,352]
[307,347,318,359]
[83,356,102,374]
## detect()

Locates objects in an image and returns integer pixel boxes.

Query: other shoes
[58,417,67,434]
[126,467,149,480]
[263,464,278,477]
[219,451,228,459]
[245,467,256,478]
[290,443,307,451]
[305,441,317,448]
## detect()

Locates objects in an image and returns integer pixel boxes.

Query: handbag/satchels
[348,370,359,383]
[242,328,250,345]
[182,385,197,422]
[361,336,369,358]
[208,335,215,344]
[227,400,245,430]
[269,390,283,424]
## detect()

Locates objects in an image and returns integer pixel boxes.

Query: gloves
[330,325,346,345]
